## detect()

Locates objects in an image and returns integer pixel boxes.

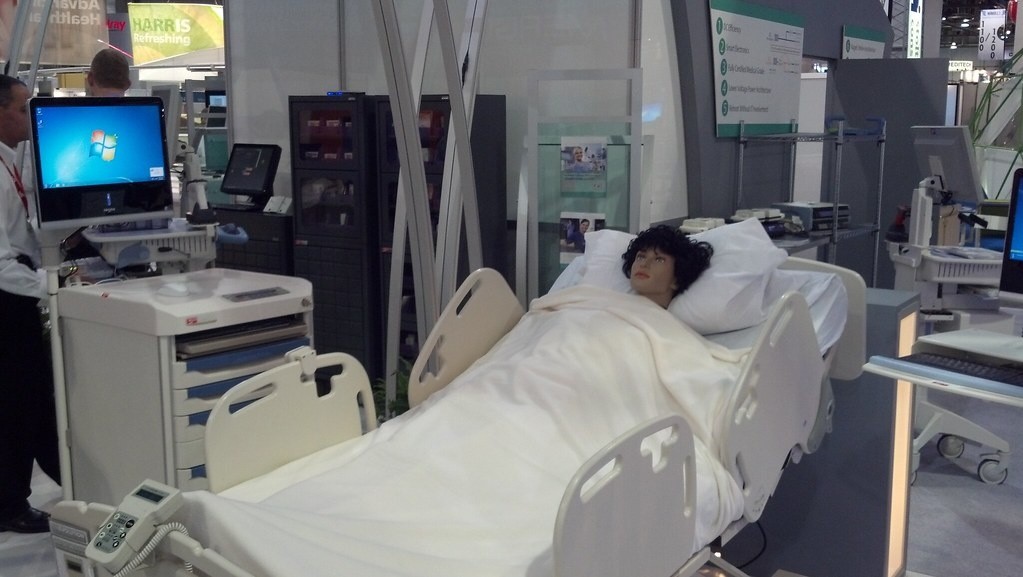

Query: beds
[49,253,867,577]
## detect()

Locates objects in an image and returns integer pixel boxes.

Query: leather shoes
[0,507,52,534]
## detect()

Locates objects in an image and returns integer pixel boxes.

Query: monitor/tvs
[27,95,174,231]
[220,143,282,211]
[910,125,984,206]
[997,167,1023,303]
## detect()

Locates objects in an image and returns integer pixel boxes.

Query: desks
[185,201,293,277]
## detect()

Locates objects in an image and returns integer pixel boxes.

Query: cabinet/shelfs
[58,266,315,508]
[738,118,889,290]
[287,95,508,407]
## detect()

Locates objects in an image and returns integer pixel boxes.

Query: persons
[89,49,132,97]
[0,75,62,533]
[622,224,714,310]
[561,219,602,253]
[574,146,607,173]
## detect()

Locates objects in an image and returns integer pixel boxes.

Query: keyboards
[898,351,1023,387]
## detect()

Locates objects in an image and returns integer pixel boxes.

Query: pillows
[582,217,787,335]
